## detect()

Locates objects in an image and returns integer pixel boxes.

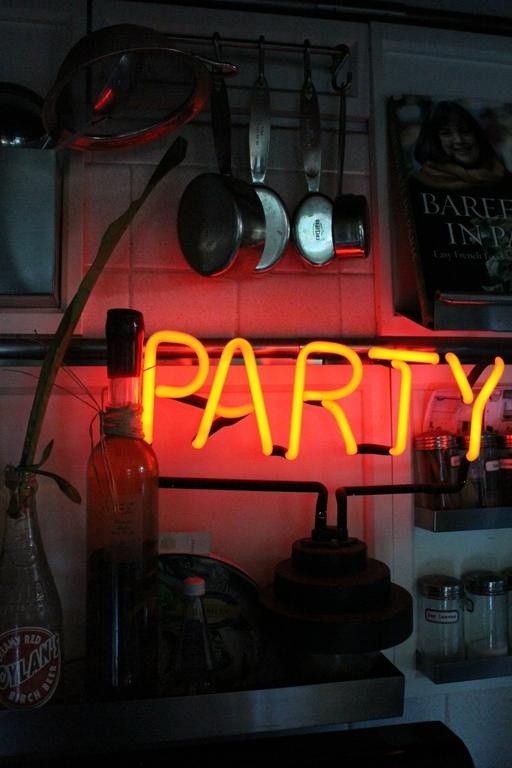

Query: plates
[157,554,272,698]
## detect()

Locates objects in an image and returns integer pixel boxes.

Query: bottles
[460,569,508,659]
[167,573,221,698]
[412,428,512,510]
[414,574,466,667]
[85,308,161,706]
[0,462,63,712]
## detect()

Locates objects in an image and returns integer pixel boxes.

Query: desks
[0,649,404,754]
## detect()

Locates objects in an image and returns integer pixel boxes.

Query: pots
[41,16,243,154]
[0,80,54,150]
[247,73,289,275]
[291,75,338,269]
[176,71,269,277]
[330,83,372,261]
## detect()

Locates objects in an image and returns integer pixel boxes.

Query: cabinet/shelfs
[415,505,511,684]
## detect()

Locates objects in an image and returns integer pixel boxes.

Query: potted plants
[0,137,188,711]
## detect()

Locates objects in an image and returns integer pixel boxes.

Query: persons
[414,102,505,190]
